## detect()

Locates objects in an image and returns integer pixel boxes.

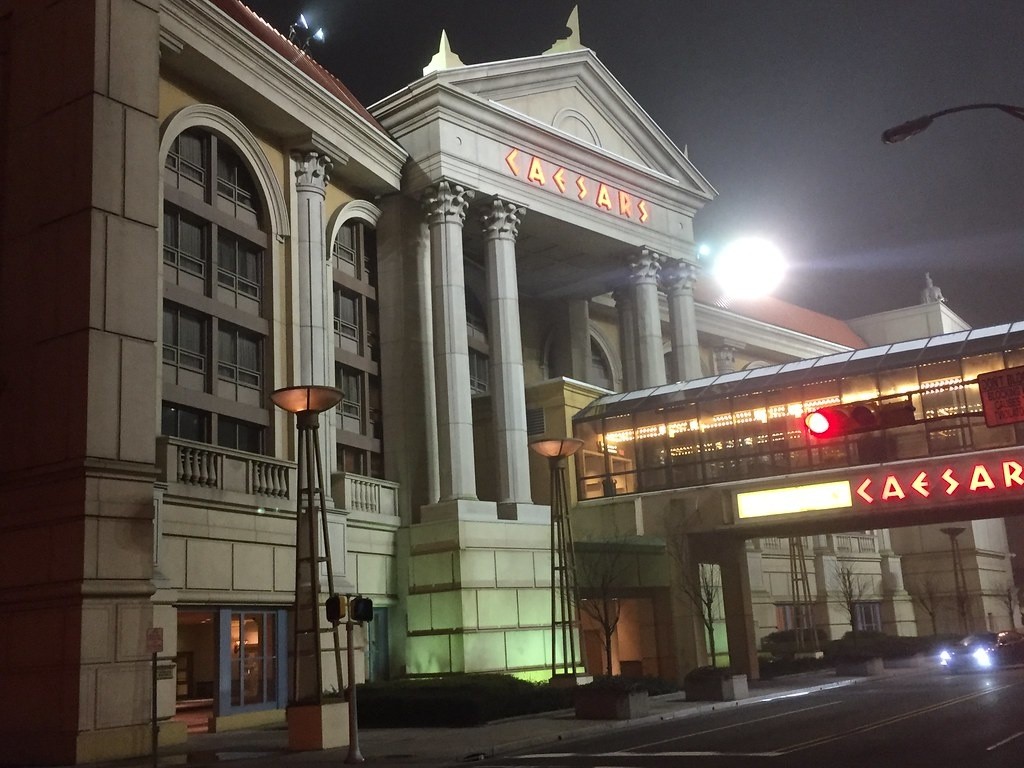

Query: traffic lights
[803,401,882,440]
[351,596,373,621]
[324,591,346,624]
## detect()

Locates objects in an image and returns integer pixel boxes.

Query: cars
[939,630,1023,672]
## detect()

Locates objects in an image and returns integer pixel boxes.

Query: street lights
[269,383,357,752]
[528,436,591,688]
[940,527,972,635]
